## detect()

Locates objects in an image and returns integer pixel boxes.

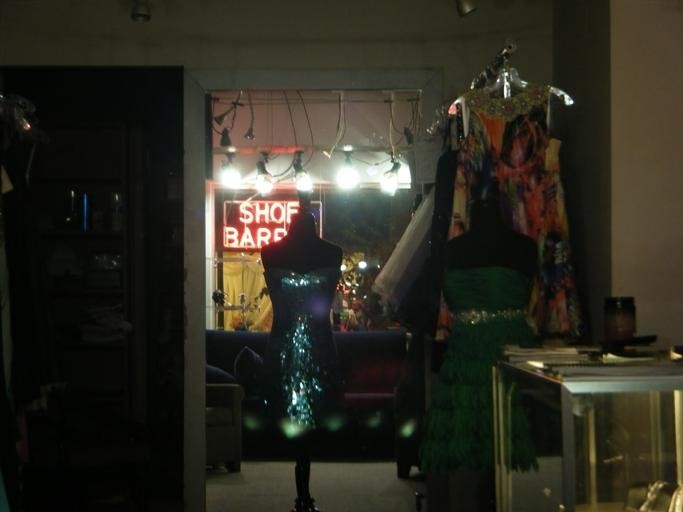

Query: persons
[261,212,342,512]
[417,177,537,478]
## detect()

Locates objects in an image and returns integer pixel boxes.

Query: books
[503,345,683,381]
